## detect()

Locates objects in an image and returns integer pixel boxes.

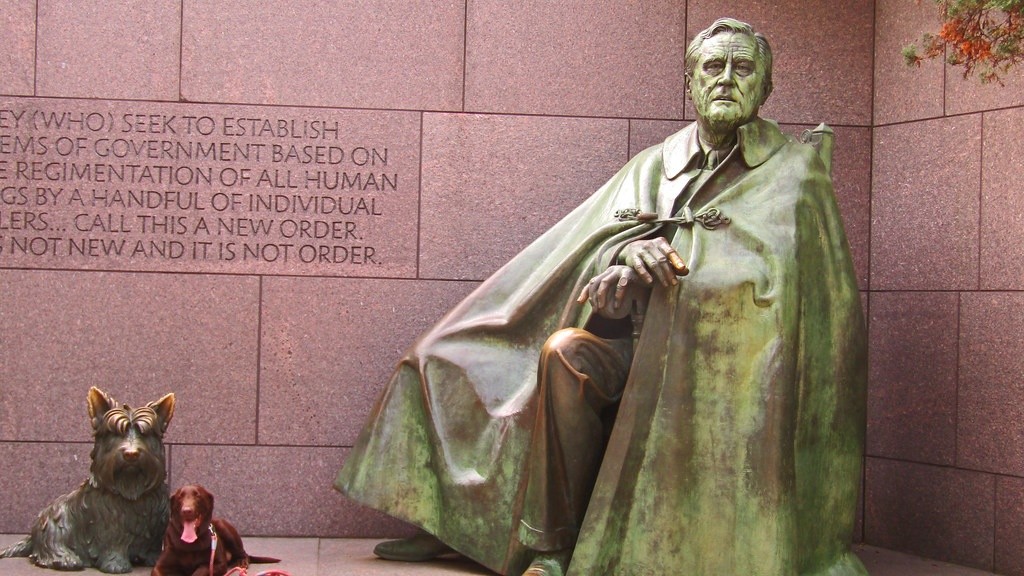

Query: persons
[331,17,870,576]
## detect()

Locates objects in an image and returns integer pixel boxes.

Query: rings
[619,276,628,286]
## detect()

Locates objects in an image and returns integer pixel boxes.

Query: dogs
[151,484,282,576]
[0,386,176,573]
